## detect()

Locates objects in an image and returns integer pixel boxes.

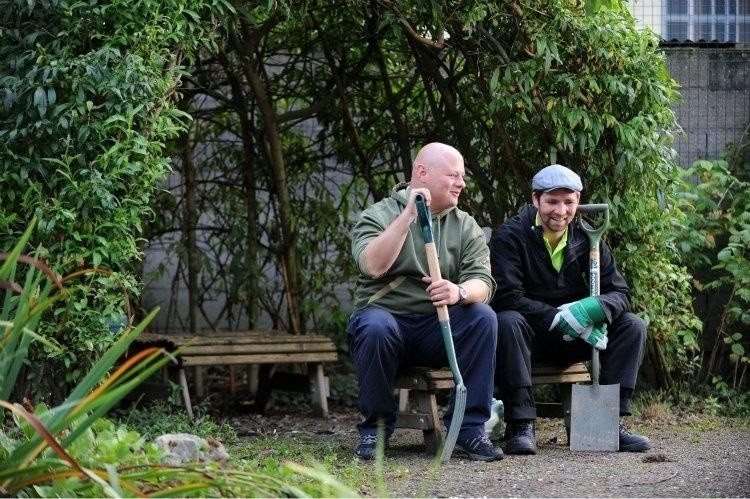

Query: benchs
[393,359,592,457]
[135,329,343,423]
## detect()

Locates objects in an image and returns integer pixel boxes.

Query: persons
[487,163,653,456]
[346,140,505,464]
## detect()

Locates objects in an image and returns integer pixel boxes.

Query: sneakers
[502,420,538,455]
[354,434,390,460]
[453,435,504,462]
[619,423,652,451]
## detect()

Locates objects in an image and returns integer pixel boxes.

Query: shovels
[569,204,620,453]
[413,193,468,466]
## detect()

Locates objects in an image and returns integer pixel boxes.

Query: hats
[531,164,584,195]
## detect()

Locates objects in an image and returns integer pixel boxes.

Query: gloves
[579,321,609,350]
[548,295,607,342]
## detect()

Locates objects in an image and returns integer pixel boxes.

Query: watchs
[457,282,467,303]
